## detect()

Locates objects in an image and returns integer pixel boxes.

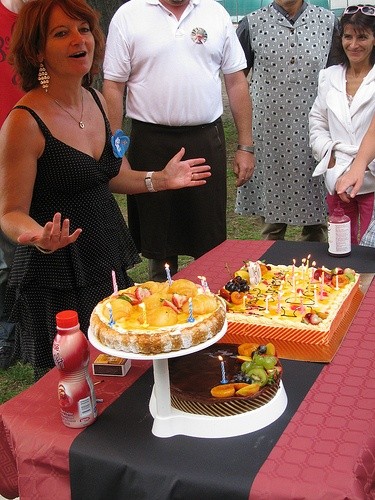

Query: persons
[336,113,375,247]
[102,0,254,281]
[307,5,375,245]
[237,1,340,241]
[0,0,211,383]
[0,0,34,371]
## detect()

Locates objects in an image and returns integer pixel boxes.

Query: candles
[188,297,195,322]
[140,303,148,326]
[243,254,339,315]
[198,276,210,292]
[218,355,225,383]
[164,262,172,286]
[111,270,118,294]
[106,302,114,325]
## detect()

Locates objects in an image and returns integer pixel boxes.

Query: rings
[192,175,193,179]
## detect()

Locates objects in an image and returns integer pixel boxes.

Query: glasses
[343,5,375,17]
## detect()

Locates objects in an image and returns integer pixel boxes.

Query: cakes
[217,254,360,344]
[91,263,226,354]
[169,342,283,417]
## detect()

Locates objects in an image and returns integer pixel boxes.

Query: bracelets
[145,171,156,192]
[35,245,54,254]
[237,144,254,154]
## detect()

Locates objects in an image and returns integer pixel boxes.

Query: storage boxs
[92,353,132,376]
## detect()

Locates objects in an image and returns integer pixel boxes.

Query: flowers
[111,129,131,159]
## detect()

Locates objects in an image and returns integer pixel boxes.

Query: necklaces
[45,90,85,128]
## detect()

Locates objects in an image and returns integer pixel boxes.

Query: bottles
[52,311,97,429]
[327,197,352,257]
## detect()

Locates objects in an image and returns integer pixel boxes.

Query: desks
[0,238,375,500]
